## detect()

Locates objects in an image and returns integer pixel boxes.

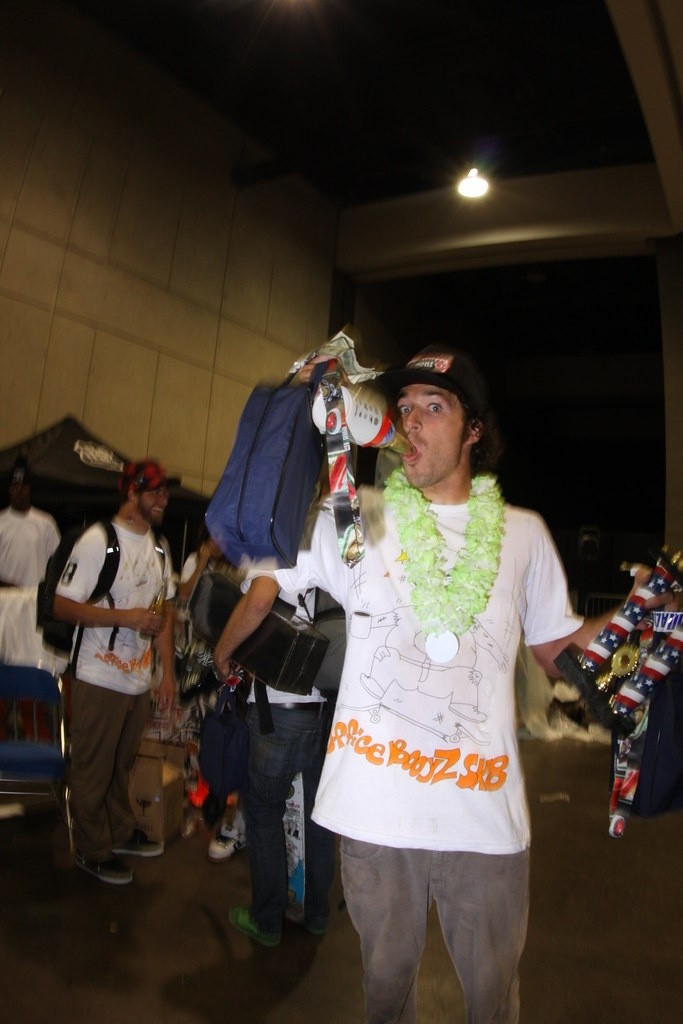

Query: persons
[178,523,347,955]
[0,463,62,588]
[272,351,683,1024]
[55,460,180,883]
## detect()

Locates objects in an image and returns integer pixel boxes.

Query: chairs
[0,664,76,858]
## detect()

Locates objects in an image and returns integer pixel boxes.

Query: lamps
[458,168,489,197]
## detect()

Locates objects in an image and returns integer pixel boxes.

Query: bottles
[140,577,170,640]
[313,385,417,456]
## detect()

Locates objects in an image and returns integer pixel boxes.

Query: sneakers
[112,830,163,857]
[228,905,282,947]
[301,919,326,935]
[74,853,133,884]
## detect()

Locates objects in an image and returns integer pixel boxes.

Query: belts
[269,702,327,711]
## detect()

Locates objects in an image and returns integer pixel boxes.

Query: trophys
[553,544,683,742]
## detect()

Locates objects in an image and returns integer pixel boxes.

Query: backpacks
[311,584,347,694]
[35,518,166,658]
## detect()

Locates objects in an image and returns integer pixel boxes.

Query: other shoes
[208,824,246,863]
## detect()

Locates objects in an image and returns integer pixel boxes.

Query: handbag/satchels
[205,355,325,570]
[197,682,251,796]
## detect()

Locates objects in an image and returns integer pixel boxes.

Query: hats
[118,458,181,494]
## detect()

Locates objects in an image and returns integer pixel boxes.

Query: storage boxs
[186,569,330,695]
[129,740,185,845]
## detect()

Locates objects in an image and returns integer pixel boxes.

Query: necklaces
[383,464,506,664]
